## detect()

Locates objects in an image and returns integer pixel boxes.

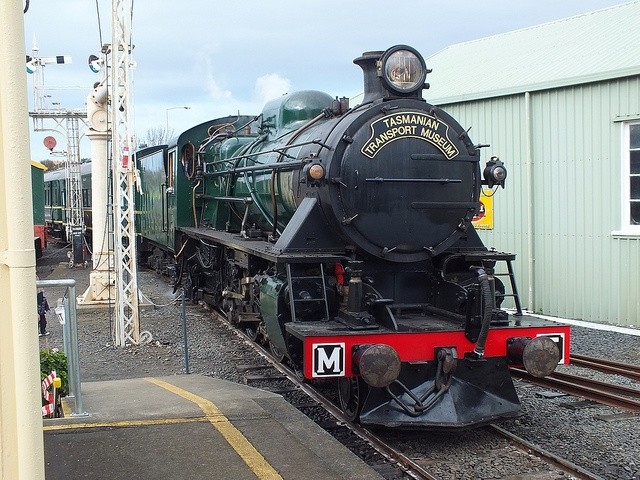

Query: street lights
[166,107,191,131]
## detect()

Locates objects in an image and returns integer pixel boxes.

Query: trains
[45,44,571,428]
